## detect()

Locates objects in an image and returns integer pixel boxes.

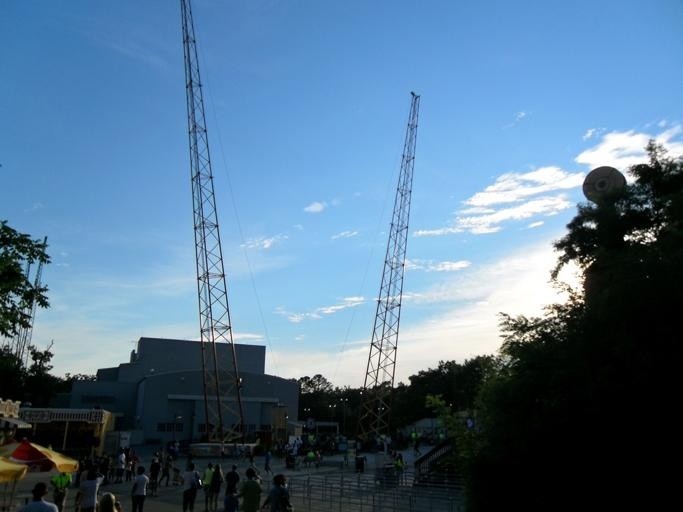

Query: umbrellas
[0,455,27,486]
[0,436,78,512]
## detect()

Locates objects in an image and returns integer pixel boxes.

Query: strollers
[172,467,185,486]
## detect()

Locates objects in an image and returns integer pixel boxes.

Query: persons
[71,444,294,512]
[48,468,73,512]
[276,427,444,486]
[18,481,60,512]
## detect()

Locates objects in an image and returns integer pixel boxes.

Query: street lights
[329,403,336,422]
[450,403,452,417]
[378,407,385,431]
[304,407,311,421]
[360,388,366,397]
[341,398,349,434]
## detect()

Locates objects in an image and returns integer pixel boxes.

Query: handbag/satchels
[194,478,203,489]
